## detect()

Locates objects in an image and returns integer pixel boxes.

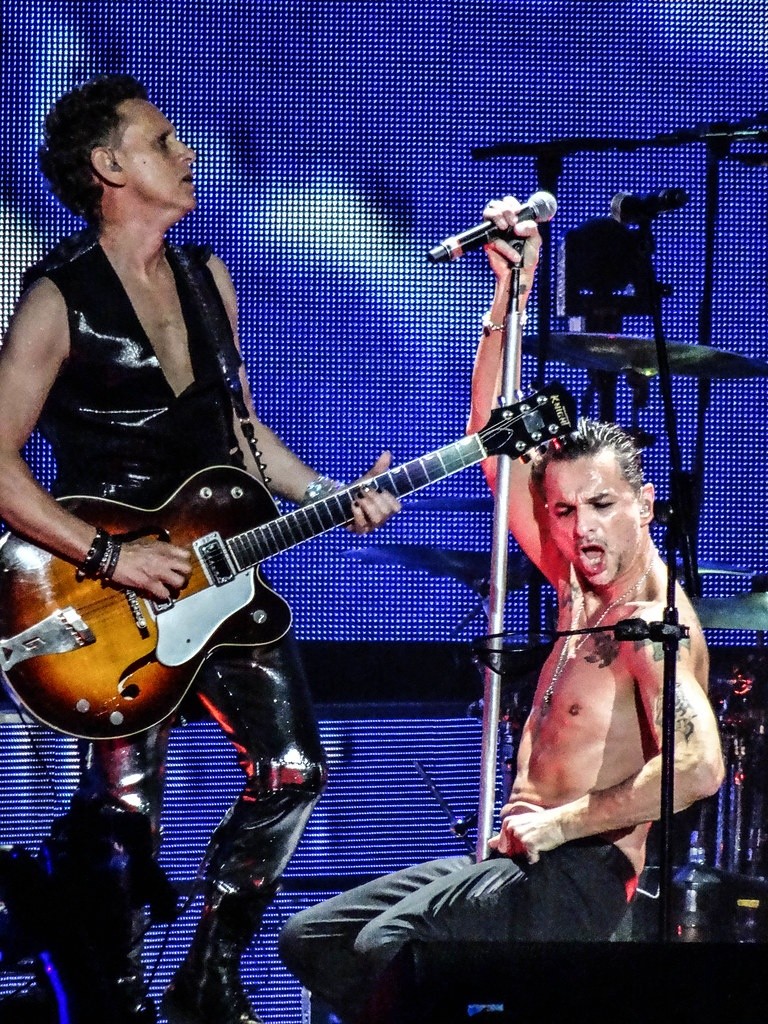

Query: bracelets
[301,475,349,508]
[478,308,527,335]
[74,524,121,587]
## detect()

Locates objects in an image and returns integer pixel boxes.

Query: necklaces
[539,548,661,718]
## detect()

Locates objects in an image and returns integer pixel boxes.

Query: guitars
[0,381,579,740]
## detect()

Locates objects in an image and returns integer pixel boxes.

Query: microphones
[427,191,558,264]
[609,187,688,222]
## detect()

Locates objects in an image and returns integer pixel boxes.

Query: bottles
[672,829,725,931]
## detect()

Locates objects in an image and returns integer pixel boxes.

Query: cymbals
[338,547,529,579]
[662,558,751,575]
[690,592,768,631]
[522,333,768,380]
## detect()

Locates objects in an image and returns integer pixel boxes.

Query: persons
[4,72,401,1021]
[277,189,734,1023]
